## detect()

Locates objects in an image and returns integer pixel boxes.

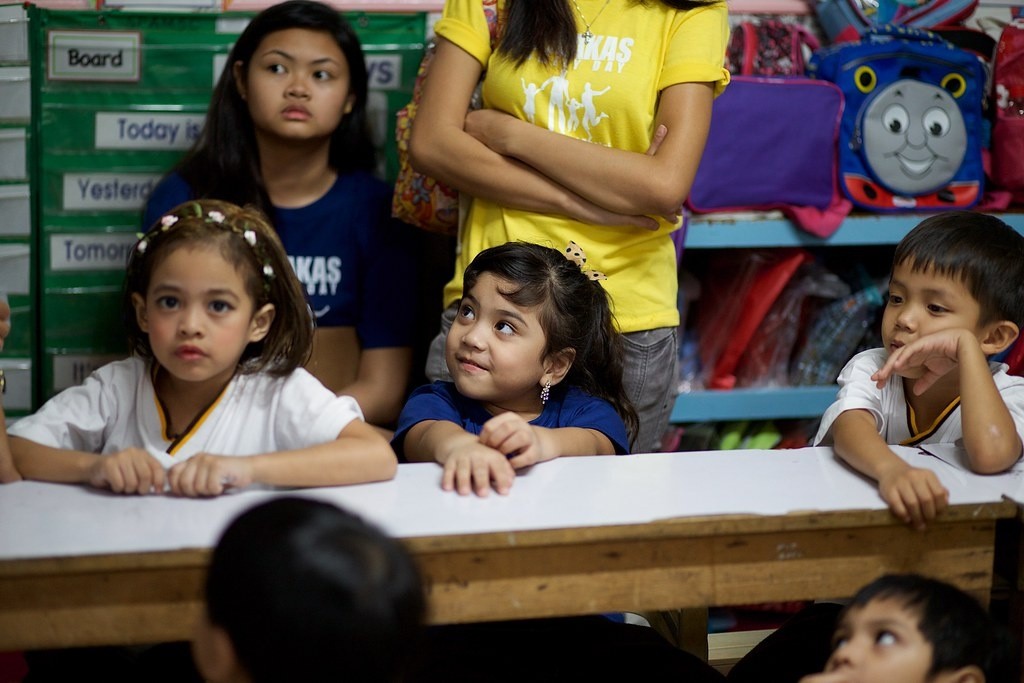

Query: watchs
[0,369,6,394]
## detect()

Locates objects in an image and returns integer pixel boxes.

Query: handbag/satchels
[390,0,500,236]
[676,243,895,387]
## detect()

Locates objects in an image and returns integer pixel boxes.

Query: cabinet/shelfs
[669,211,1023,423]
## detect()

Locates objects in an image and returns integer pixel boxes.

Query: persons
[798,574,988,683]
[726,211,1024,683]
[407,0,732,453]
[140,0,413,424]
[192,497,428,683]
[389,240,727,683]
[6,199,398,683]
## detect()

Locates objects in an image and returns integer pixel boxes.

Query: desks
[0,447,1018,661]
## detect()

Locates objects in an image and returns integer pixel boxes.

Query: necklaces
[573,0,609,43]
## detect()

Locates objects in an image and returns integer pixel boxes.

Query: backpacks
[813,0,979,47]
[972,17,1024,213]
[806,22,986,212]
[684,22,853,240]
